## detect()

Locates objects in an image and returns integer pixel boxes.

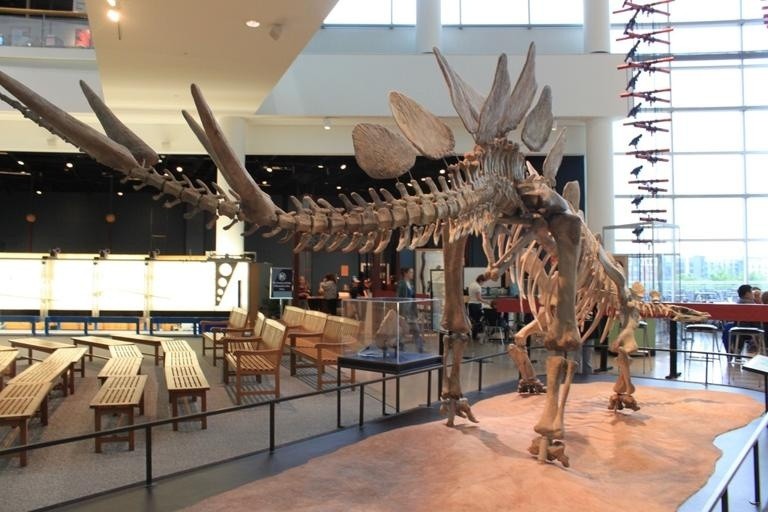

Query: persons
[735,285,768,348]
[296,272,386,315]
[468,274,491,340]
[488,287,514,342]
[398,267,425,354]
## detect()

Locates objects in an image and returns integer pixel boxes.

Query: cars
[644,286,739,303]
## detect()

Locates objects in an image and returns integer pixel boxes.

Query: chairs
[681,320,721,363]
[200,306,364,405]
[617,321,653,374]
[728,320,766,363]
[483,307,511,345]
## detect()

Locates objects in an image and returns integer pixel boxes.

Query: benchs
[0,333,211,466]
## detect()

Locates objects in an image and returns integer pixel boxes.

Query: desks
[337,352,444,427]
